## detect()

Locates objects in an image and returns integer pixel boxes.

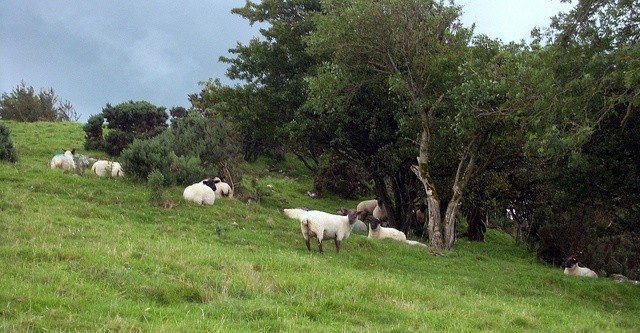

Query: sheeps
[300,208,363,254]
[199,174,233,199]
[91,160,122,177]
[111,164,125,177]
[51,148,76,173]
[357,198,378,220]
[366,216,406,241]
[415,201,427,223]
[373,195,388,221]
[183,178,220,205]
[563,256,598,278]
[336,206,367,231]
